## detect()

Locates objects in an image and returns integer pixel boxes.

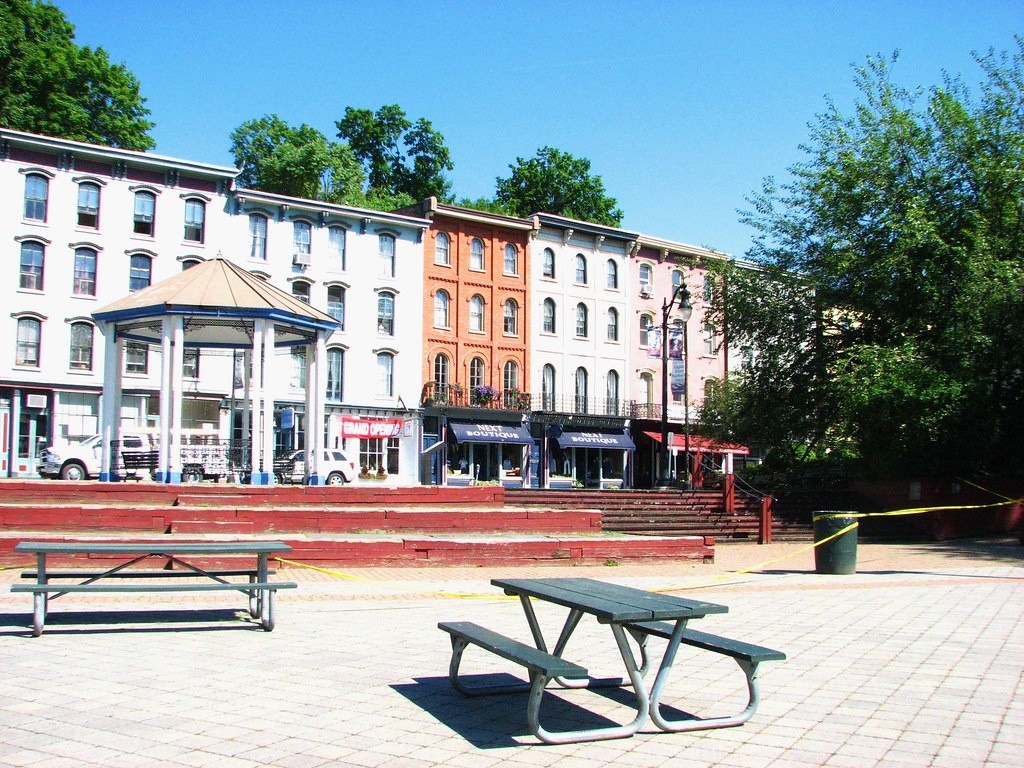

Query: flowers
[361,466,368,474]
[451,383,464,392]
[377,466,385,475]
[476,385,500,402]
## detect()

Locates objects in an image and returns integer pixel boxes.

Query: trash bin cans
[812,510,858,575]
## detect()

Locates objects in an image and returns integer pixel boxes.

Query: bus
[116,427,227,483]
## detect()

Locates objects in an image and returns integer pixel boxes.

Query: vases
[358,473,370,478]
[376,474,387,479]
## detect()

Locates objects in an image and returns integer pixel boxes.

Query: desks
[449,577,759,745]
[15,541,293,635]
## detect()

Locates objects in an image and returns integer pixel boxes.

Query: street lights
[655,283,694,486]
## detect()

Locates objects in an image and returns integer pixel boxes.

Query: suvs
[35,432,152,480]
[272,449,358,488]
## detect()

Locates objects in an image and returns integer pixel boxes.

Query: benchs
[19,570,277,616]
[627,621,786,725]
[121,450,159,483]
[260,458,297,484]
[438,621,589,745]
[11,582,298,631]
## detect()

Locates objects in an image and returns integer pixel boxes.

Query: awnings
[448,421,535,445]
[642,431,750,454]
[557,432,636,450]
